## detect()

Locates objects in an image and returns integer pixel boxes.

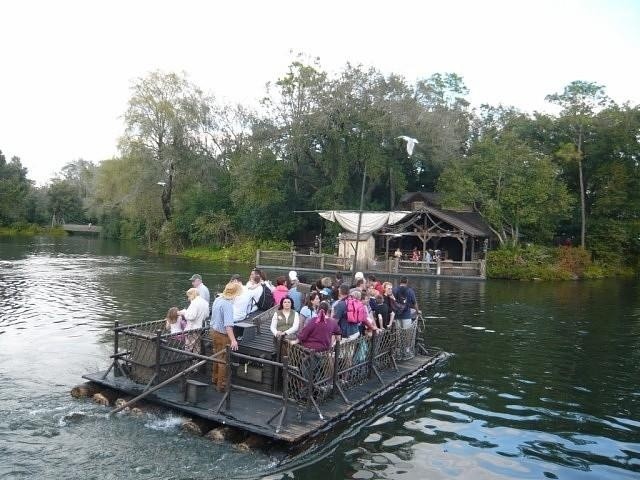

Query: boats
[71,165,444,462]
[64,230,100,235]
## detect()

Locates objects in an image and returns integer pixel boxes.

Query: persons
[166,267,422,399]
[394,248,403,261]
[409,246,441,262]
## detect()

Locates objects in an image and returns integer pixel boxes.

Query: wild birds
[156,181,166,186]
[393,135,419,159]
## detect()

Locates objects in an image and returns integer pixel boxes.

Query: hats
[189,274,202,282]
[289,271,297,282]
[355,272,363,280]
